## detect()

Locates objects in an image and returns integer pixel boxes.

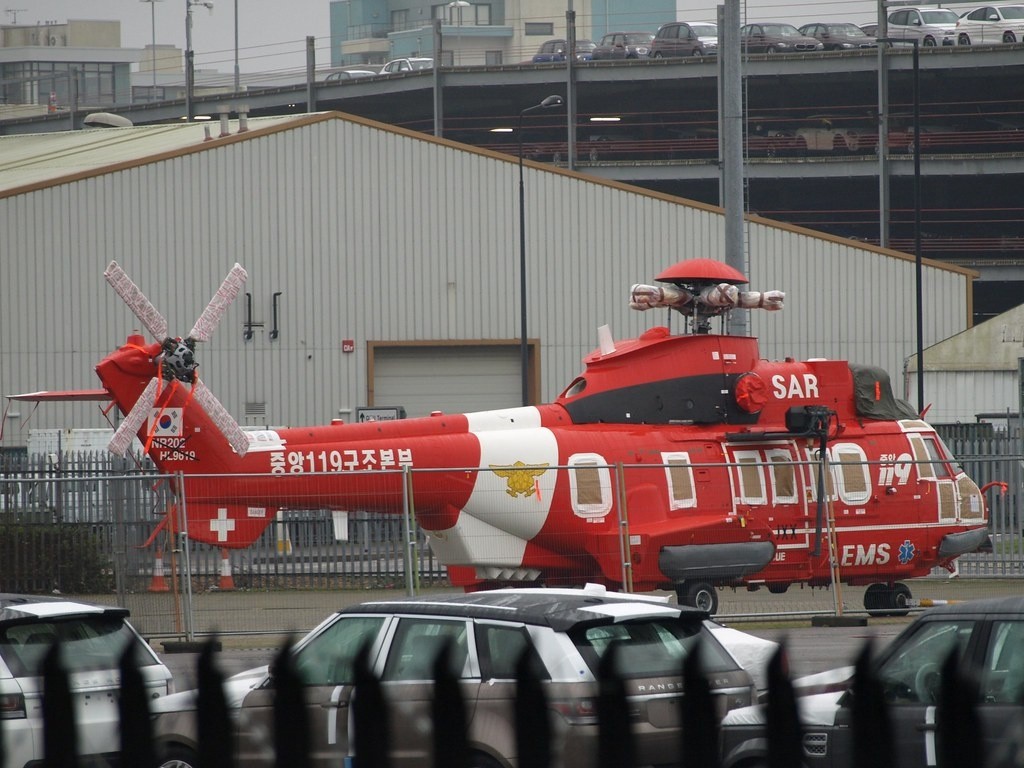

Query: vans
[818,598,1024,768]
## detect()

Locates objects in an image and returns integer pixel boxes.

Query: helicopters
[7,259,1012,630]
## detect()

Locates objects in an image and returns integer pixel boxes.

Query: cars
[707,594,1024,768]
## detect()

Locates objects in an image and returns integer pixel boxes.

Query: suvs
[148,584,785,768]
[0,592,187,768]
